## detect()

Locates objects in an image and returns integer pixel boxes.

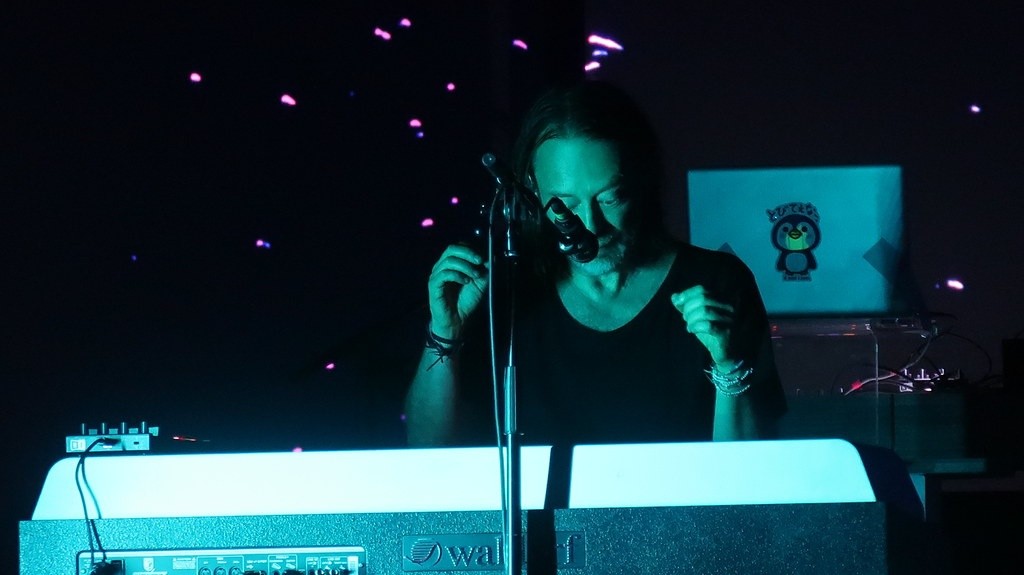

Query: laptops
[685,166,909,315]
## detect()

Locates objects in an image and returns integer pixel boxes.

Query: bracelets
[703,360,754,396]
[424,320,460,369]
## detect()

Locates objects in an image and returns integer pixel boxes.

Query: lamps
[937,256,976,296]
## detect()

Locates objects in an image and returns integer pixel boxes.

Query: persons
[404,80,788,448]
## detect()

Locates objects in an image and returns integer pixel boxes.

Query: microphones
[550,197,599,263]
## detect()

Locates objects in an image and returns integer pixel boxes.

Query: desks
[769,310,953,445]
